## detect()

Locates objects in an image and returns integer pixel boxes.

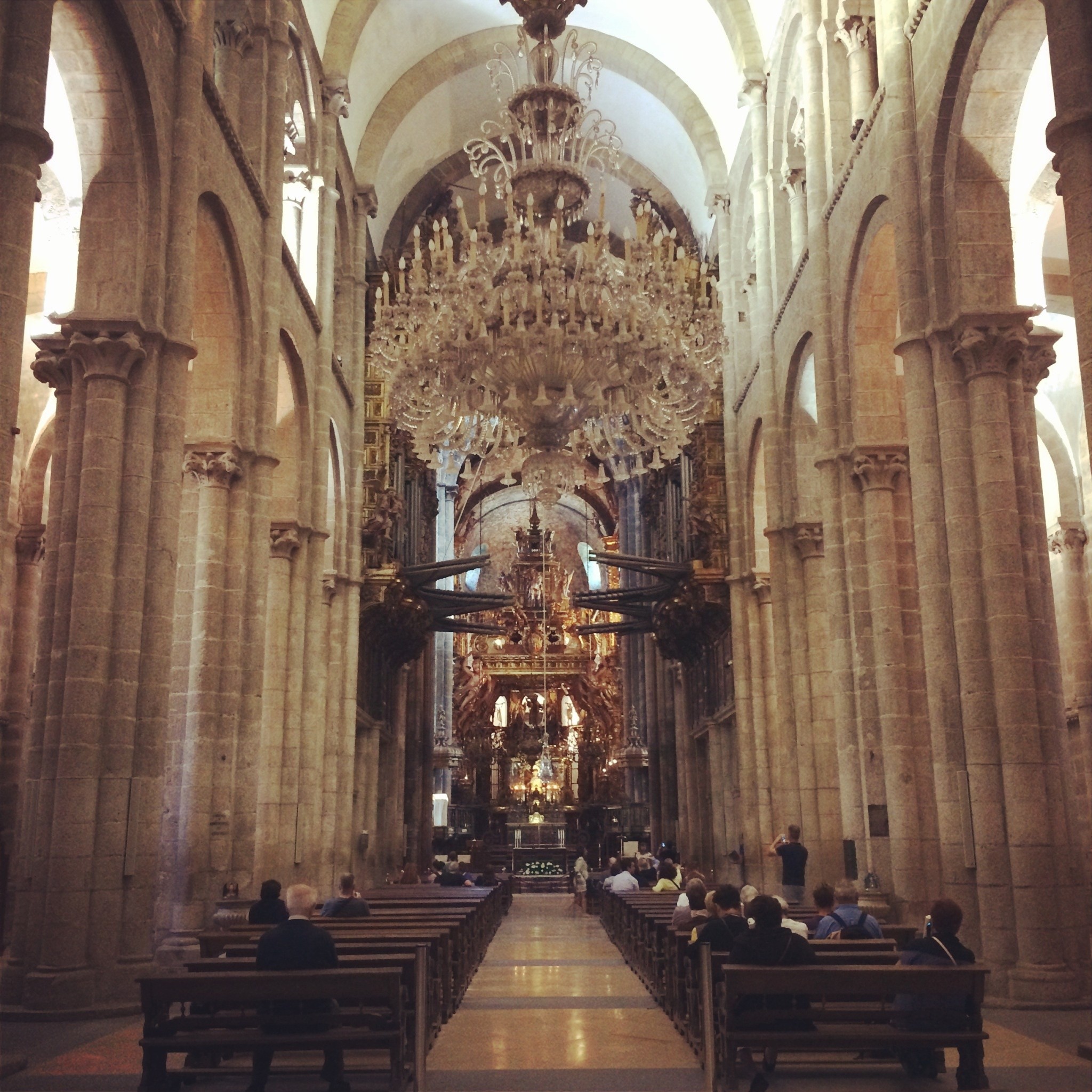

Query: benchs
[133,882,990,1092]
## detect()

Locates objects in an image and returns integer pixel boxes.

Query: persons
[400,851,505,888]
[727,897,819,1090]
[572,846,590,918]
[677,869,885,947]
[247,885,348,1092]
[321,873,372,918]
[248,880,289,925]
[891,899,987,1092]
[600,842,685,892]
[768,824,808,902]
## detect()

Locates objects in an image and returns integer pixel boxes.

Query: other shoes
[329,1081,351,1092]
[246,1083,265,1091]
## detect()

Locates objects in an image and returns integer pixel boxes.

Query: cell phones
[662,841,665,848]
[782,837,786,844]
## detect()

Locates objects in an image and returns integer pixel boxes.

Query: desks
[506,823,568,847]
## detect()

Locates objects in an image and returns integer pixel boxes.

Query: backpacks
[826,912,877,965]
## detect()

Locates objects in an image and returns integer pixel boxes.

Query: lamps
[537,529,553,784]
[367,0,732,510]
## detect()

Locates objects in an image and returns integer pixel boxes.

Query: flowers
[519,862,564,874]
